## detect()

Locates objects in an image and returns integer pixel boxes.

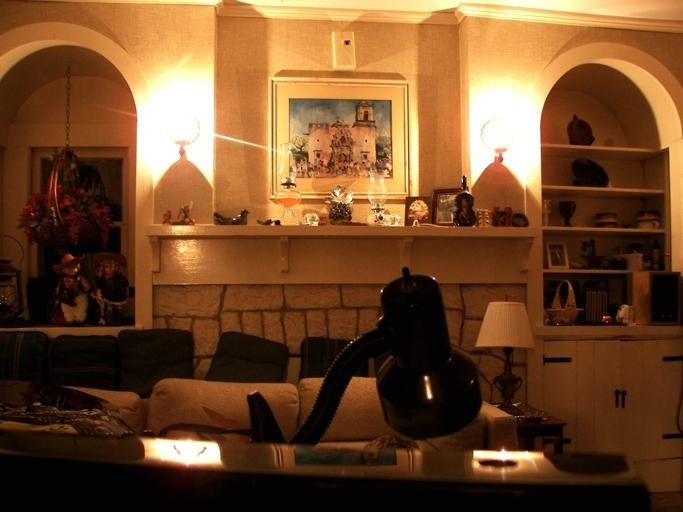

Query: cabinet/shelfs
[539,142,683,494]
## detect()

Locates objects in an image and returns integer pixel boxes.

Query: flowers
[15,148,112,246]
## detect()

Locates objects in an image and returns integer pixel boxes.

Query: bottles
[453,175,476,226]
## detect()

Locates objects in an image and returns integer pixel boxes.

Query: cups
[638,210,661,230]
[594,212,617,228]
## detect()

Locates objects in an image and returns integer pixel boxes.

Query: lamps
[0,233,26,325]
[287,265,482,446]
[474,301,536,416]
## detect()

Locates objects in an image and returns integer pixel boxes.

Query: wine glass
[558,201,575,227]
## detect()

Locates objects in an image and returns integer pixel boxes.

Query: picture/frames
[432,188,462,226]
[267,76,410,199]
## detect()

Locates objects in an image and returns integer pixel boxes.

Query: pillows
[0,379,485,438]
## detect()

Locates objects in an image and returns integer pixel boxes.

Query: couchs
[0,325,518,478]
